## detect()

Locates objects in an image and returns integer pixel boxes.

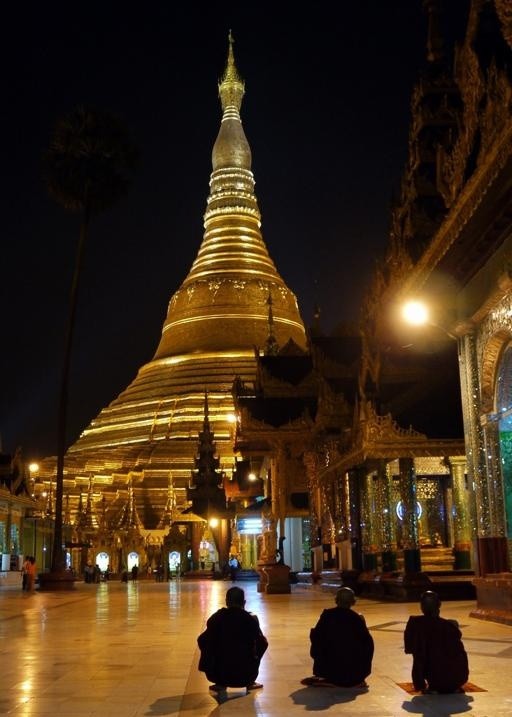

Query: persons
[175,563,181,581]
[404,589,470,695]
[120,572,128,583]
[144,560,172,582]
[197,587,268,690]
[85,564,110,584]
[229,555,238,581]
[309,587,374,687]
[132,564,139,584]
[223,564,229,580]
[200,556,205,569]
[21,556,36,593]
